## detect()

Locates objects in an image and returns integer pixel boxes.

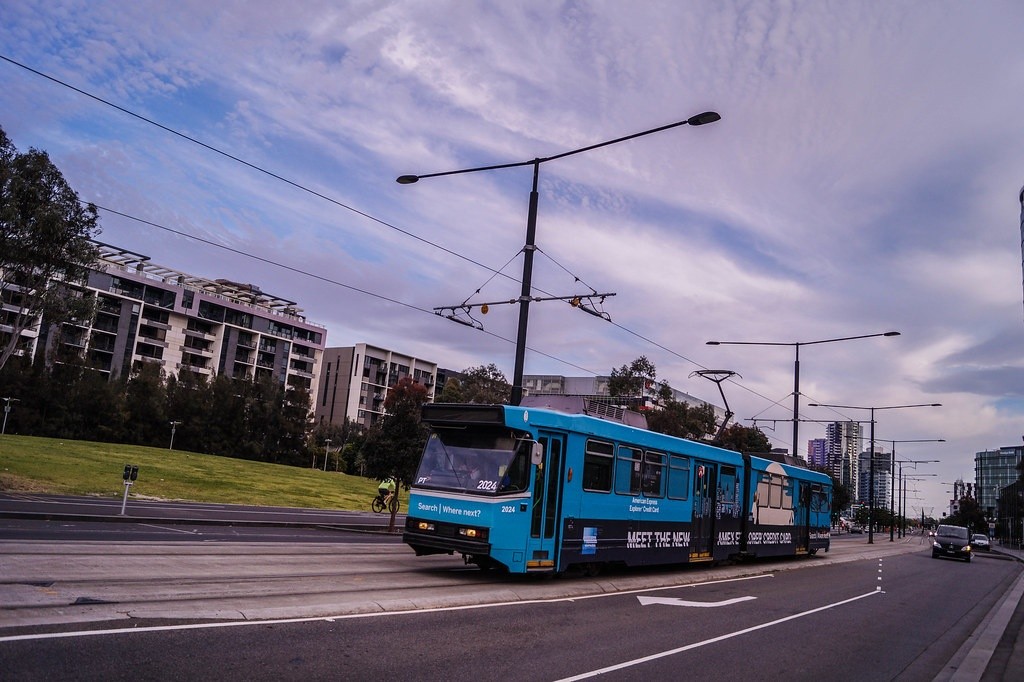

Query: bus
[401,404,833,580]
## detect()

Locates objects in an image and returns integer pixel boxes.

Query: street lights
[395,112,722,407]
[872,459,940,542]
[881,471,939,539]
[809,403,941,544]
[844,434,946,542]
[706,332,901,458]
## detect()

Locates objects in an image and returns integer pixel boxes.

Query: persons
[377,476,397,509]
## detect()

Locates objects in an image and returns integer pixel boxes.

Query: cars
[933,525,971,559]
[971,535,990,552]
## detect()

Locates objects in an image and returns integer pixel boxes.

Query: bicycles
[372,491,400,513]
